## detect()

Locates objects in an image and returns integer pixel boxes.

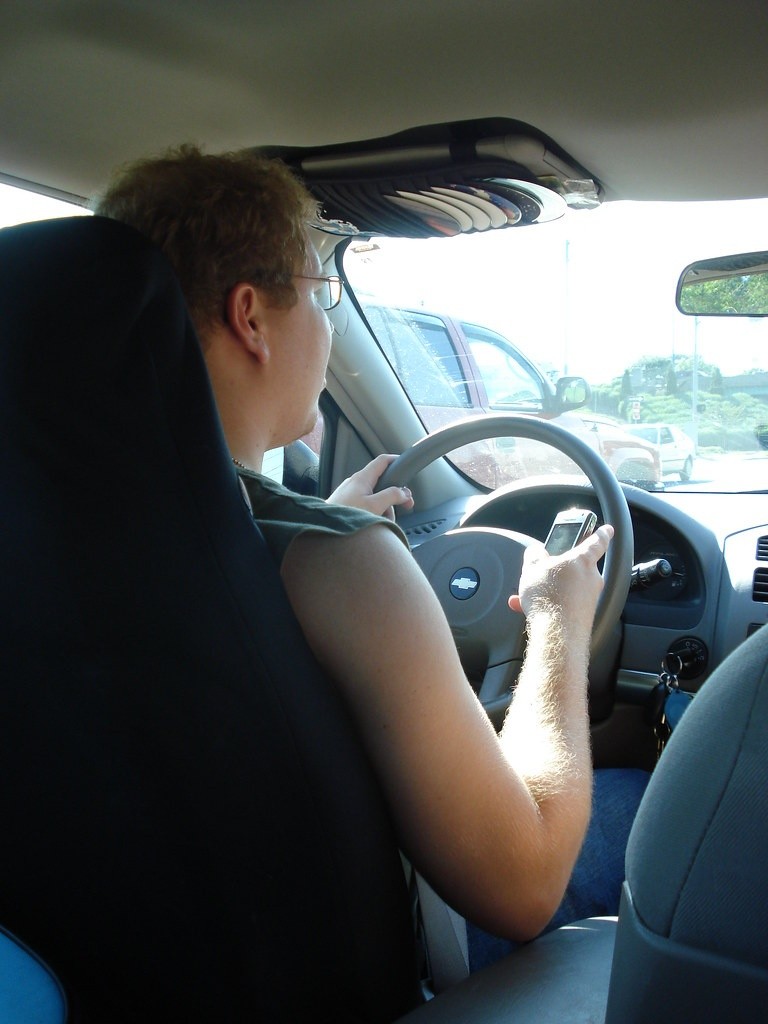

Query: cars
[622,422,697,482]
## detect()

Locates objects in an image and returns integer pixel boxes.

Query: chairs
[0,215,768,1024]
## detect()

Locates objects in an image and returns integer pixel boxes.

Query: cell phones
[544,509,597,556]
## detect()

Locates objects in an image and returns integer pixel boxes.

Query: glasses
[261,275,345,311]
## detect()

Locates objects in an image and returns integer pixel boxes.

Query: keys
[643,648,694,760]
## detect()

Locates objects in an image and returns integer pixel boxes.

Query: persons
[90,148,655,982]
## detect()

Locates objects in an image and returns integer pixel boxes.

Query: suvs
[295,296,666,491]
